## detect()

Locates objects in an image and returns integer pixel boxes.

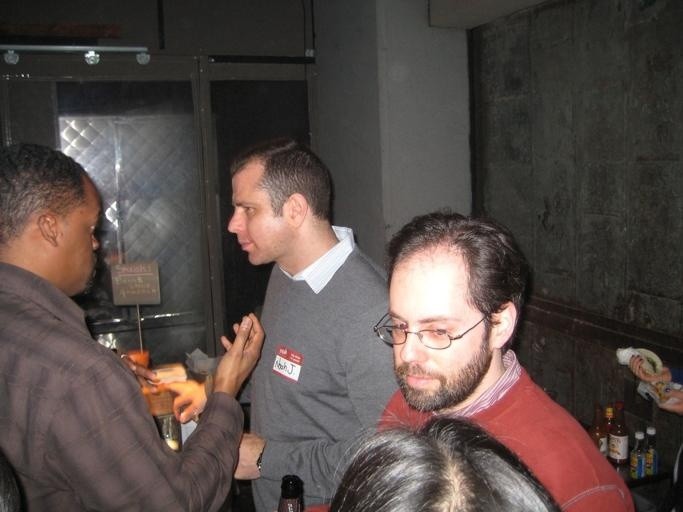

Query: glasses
[372,312,488,350]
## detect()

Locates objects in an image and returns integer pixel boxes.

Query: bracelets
[256,445,263,474]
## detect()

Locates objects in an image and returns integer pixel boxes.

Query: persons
[318,413,560,512]
[1,140,269,509]
[627,350,682,417]
[372,203,639,510]
[145,134,402,510]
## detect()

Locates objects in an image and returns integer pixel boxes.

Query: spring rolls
[616,347,663,377]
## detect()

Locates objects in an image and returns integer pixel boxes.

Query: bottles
[593,399,659,481]
